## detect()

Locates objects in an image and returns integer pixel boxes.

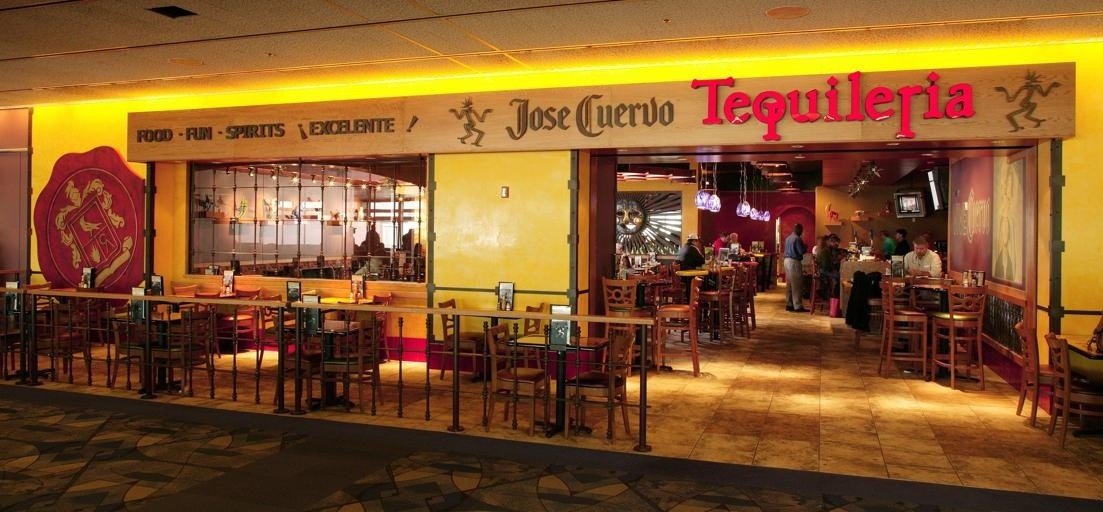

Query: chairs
[599,246,776,377]
[1047,334,1102,447]
[1,275,396,414]
[809,245,988,394]
[1012,321,1059,427]
[440,298,639,440]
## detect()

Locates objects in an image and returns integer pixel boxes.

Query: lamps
[692,163,771,223]
[222,164,410,196]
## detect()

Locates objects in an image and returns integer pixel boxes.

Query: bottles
[499,294,510,311]
[221,279,226,293]
[891,261,904,278]
[885,260,891,276]
[353,287,358,300]
[962,270,977,288]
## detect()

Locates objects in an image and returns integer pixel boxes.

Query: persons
[969,278,978,287]
[499,292,510,310]
[672,225,942,312]
[351,223,423,280]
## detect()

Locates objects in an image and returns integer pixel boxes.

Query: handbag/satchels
[829,297,842,317]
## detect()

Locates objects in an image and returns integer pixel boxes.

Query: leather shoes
[786,306,810,312]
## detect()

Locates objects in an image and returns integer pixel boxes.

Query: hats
[688,234,704,243]
[828,234,839,241]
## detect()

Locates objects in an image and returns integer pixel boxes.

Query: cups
[750,257,755,262]
[727,259,732,267]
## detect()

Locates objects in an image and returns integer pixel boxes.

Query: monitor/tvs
[894,192,927,218]
